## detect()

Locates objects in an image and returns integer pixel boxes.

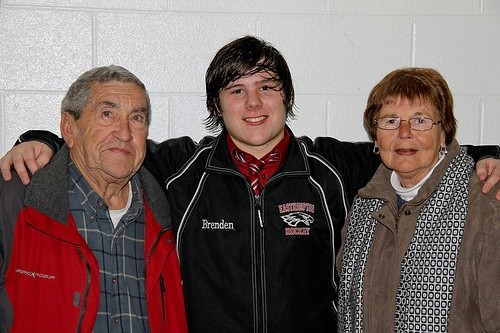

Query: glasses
[374,116,442,131]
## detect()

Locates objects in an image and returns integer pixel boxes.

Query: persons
[1,37,499,333]
[336,68,500,332]
[0,65,187,333]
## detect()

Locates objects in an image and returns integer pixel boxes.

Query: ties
[233,149,281,196]
